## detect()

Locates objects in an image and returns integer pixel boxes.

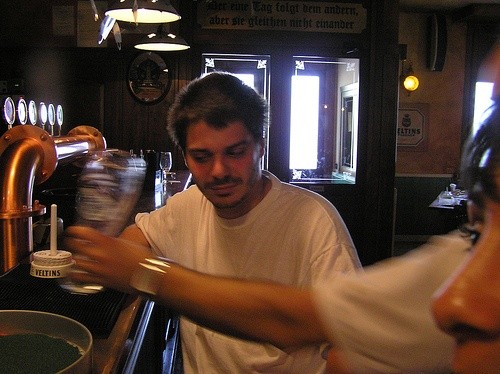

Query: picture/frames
[125,49,172,106]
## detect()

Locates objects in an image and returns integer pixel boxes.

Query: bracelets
[129,258,171,296]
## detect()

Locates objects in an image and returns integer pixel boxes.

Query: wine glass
[159,151,172,183]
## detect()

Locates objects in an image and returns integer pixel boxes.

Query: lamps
[105,0,191,52]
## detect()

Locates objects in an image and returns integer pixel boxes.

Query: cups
[450,184,456,194]
[61,148,146,295]
[343,172,352,182]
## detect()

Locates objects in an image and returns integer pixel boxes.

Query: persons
[62,72,500,374]
[115,72,365,374]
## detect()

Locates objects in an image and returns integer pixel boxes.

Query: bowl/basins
[0,310,93,374]
